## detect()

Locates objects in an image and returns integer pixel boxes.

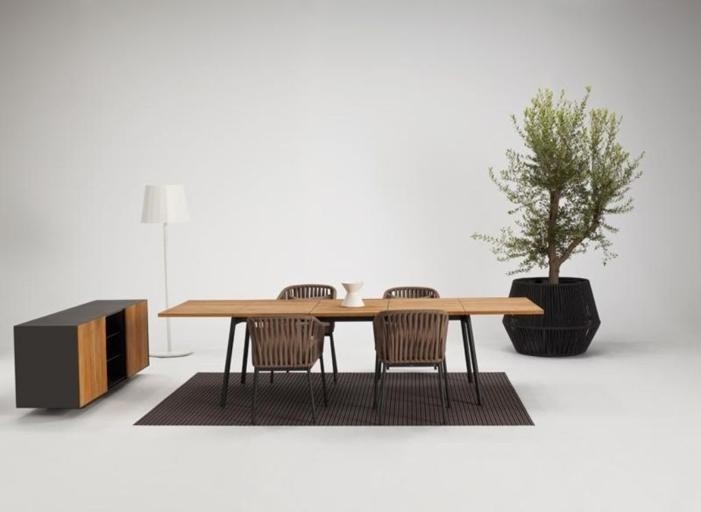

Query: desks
[158,295,547,409]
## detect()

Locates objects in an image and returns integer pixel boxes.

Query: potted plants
[467,82,648,359]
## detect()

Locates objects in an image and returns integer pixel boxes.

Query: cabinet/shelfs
[13,297,149,412]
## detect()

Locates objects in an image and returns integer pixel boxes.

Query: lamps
[140,181,193,359]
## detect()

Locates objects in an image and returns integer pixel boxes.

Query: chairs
[246,282,454,426]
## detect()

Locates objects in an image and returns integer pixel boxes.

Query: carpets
[132,369,537,428]
